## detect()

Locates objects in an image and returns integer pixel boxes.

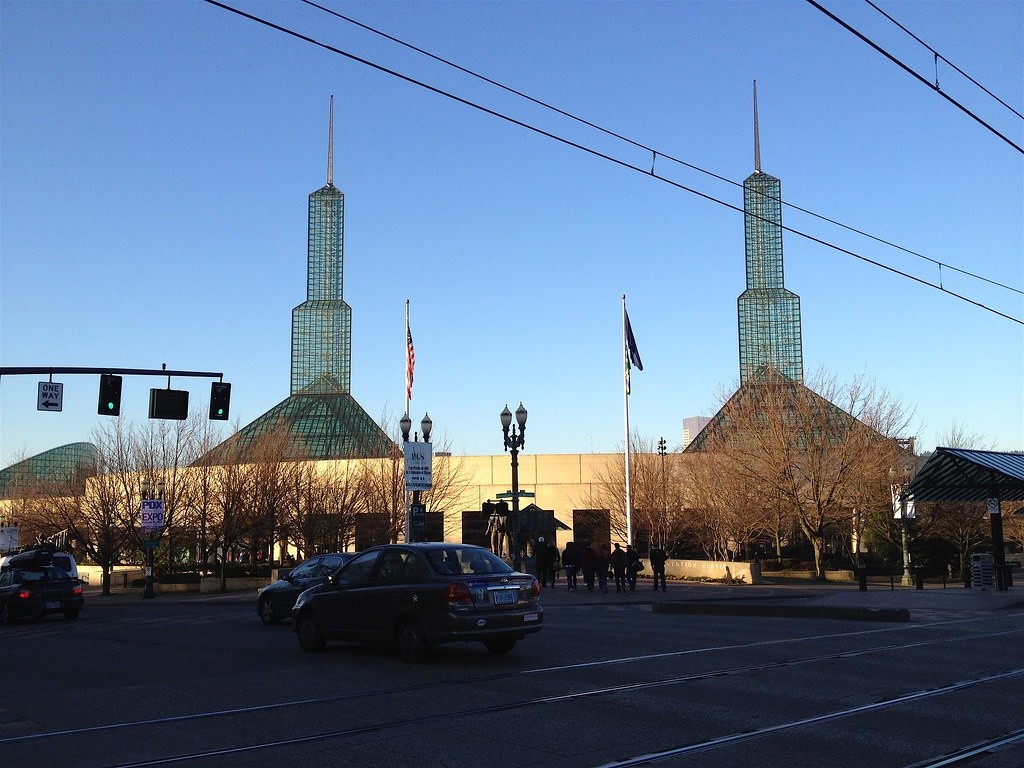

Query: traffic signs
[98,374,122,416]
[37,381,63,411]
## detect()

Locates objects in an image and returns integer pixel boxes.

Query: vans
[0,553,78,581]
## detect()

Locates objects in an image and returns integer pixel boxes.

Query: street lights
[400,412,432,542]
[888,463,914,586]
[656,440,668,546]
[140,475,165,598]
[500,402,527,572]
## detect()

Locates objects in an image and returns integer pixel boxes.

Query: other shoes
[569,588,574,592]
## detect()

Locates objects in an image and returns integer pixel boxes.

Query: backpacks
[637,561,644,571]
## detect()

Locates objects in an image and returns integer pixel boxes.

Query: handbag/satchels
[607,570,613,579]
[553,560,562,571]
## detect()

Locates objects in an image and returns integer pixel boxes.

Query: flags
[625,345,631,396]
[623,309,643,371]
[405,322,415,401]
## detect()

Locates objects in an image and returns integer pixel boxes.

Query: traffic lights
[149,389,189,420]
[209,383,231,420]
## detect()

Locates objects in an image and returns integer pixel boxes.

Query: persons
[650,544,667,592]
[521,537,639,595]
[237,550,302,564]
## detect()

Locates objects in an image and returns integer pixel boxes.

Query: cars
[252,552,378,626]
[291,542,545,663]
[0,548,83,625]
